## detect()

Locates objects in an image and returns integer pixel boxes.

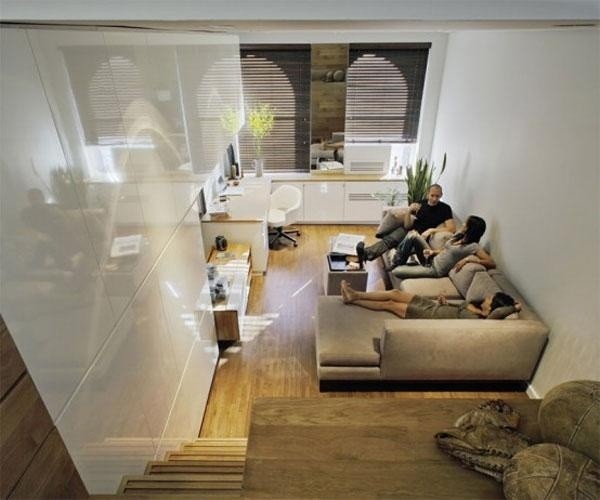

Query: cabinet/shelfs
[205,246,255,344]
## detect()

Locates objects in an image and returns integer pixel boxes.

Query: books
[222,185,245,196]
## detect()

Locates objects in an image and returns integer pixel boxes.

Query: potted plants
[222,106,245,174]
[251,105,273,174]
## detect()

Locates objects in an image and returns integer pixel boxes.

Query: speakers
[216,236,227,251]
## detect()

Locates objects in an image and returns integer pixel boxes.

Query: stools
[503,444,600,500]
[538,380,600,463]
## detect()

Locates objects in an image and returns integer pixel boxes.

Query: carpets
[240,398,545,500]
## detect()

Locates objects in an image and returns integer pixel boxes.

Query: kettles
[232,162,243,180]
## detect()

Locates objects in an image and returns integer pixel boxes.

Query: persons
[382,214,497,278]
[0,187,118,329]
[339,278,524,322]
[356,183,456,265]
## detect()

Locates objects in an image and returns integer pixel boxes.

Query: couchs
[316,205,549,392]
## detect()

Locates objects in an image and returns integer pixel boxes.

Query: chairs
[266,186,303,249]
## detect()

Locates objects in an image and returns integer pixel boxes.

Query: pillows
[468,273,499,306]
[450,255,483,288]
[427,222,458,246]
[375,209,407,230]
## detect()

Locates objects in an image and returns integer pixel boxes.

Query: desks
[202,177,272,275]
